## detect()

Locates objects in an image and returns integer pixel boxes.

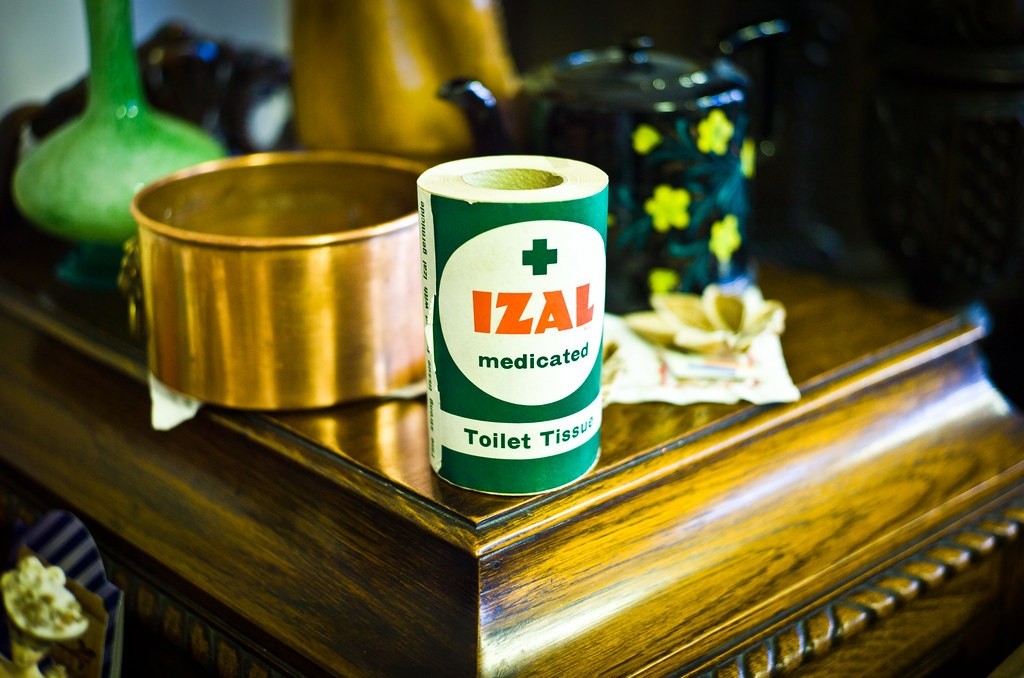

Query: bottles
[10,1,226,252]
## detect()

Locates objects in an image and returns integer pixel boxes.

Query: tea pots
[433,32,762,315]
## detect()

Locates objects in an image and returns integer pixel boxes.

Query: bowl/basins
[119,148,429,409]
